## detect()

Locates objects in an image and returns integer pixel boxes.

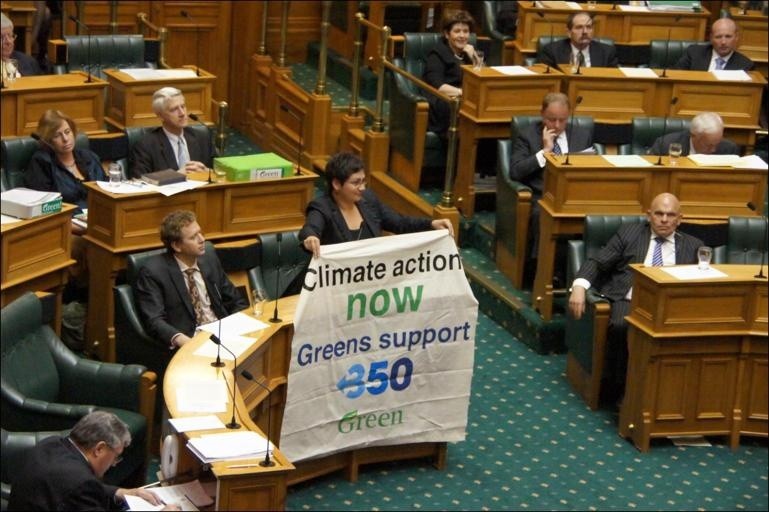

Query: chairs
[0,0,479,510]
[457,2,769,453]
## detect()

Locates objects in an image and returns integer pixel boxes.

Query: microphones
[574,12,597,74]
[210,334,242,429]
[30,132,65,207]
[180,10,201,77]
[561,96,583,165]
[208,276,225,367]
[356,221,366,240]
[660,14,681,79]
[654,97,678,167]
[537,11,554,73]
[241,369,275,468]
[280,104,304,176]
[189,113,216,184]
[69,14,94,83]
[747,201,768,279]
[269,232,282,323]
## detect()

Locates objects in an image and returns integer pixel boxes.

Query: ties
[552,140,562,156]
[714,58,725,69]
[576,52,586,67]
[184,268,210,328]
[652,237,665,266]
[177,140,187,169]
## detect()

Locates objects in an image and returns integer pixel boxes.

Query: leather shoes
[552,274,567,298]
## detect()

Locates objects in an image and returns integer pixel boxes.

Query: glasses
[106,445,122,466]
[0,32,16,41]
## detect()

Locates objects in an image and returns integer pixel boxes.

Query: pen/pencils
[132,177,147,185]
[226,464,258,469]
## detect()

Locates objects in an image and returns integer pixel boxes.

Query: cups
[588,1,596,8]
[668,143,682,165]
[251,287,268,315]
[214,165,227,183]
[738,1,749,18]
[4,59,19,88]
[570,53,581,73]
[472,51,484,71]
[698,246,712,269]
[108,163,123,187]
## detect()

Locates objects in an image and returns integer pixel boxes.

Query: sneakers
[59,301,88,329]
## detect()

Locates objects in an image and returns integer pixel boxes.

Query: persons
[670,16,754,70]
[567,192,705,409]
[509,92,593,298]
[648,110,739,153]
[533,11,618,66]
[130,86,222,179]
[421,7,493,175]
[137,206,248,346]
[280,151,454,296]
[4,409,184,511]
[23,108,128,303]
[0,12,46,76]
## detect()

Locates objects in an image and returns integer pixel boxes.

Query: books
[689,154,768,170]
[141,167,187,186]
